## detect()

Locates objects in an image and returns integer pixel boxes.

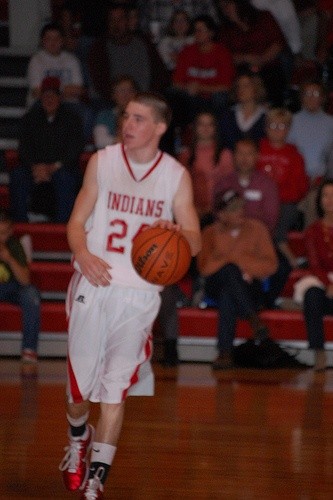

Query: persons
[300,179,333,374]
[63,92,203,500]
[195,190,280,370]
[0,208,41,380]
[157,256,193,367]
[12,0,333,310]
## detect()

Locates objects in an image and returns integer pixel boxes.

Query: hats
[214,190,241,211]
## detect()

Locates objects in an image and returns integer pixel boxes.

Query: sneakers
[80,478,104,500]
[61,425,94,493]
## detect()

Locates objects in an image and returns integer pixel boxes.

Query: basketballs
[131,227,193,285]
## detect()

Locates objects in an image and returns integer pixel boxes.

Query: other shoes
[20,349,38,378]
[154,336,180,367]
[212,351,231,369]
[260,336,281,357]
[312,351,327,372]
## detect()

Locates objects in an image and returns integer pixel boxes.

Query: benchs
[0,49,333,369]
